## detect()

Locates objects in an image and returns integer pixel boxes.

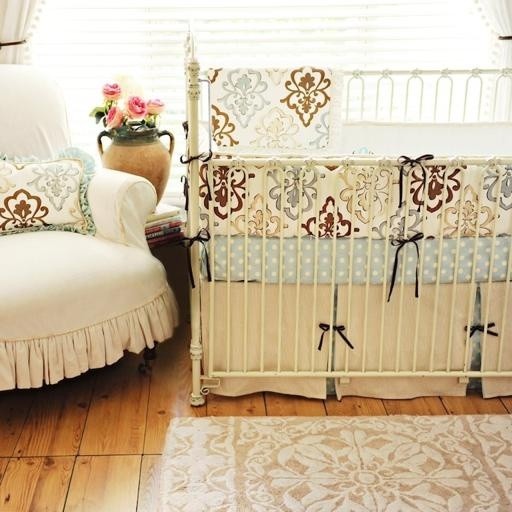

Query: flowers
[88,82,165,139]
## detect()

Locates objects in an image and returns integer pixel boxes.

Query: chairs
[0,64,182,397]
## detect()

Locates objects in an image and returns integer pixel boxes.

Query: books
[144,204,184,248]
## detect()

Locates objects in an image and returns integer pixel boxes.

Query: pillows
[0,145,98,237]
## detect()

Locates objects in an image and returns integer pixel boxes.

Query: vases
[96,127,175,214]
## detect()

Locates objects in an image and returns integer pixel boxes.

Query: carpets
[144,413,512,512]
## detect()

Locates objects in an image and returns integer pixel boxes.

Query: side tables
[149,237,190,326]
